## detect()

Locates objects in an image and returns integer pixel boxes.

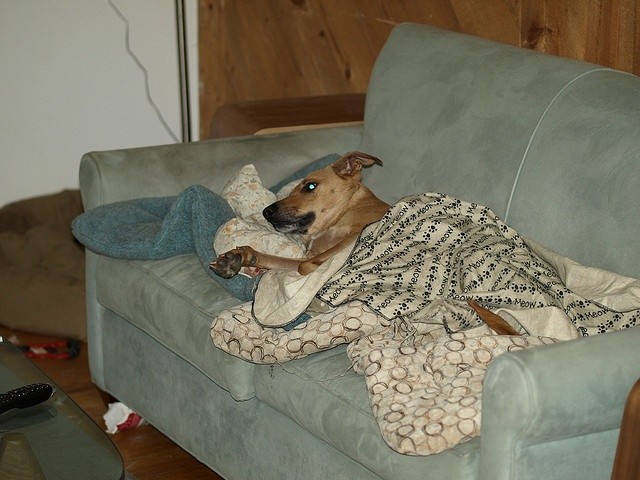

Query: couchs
[78,23,639,480]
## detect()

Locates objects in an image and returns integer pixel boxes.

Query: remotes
[0,383,53,414]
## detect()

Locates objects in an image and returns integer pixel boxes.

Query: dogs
[209,153,523,338]
